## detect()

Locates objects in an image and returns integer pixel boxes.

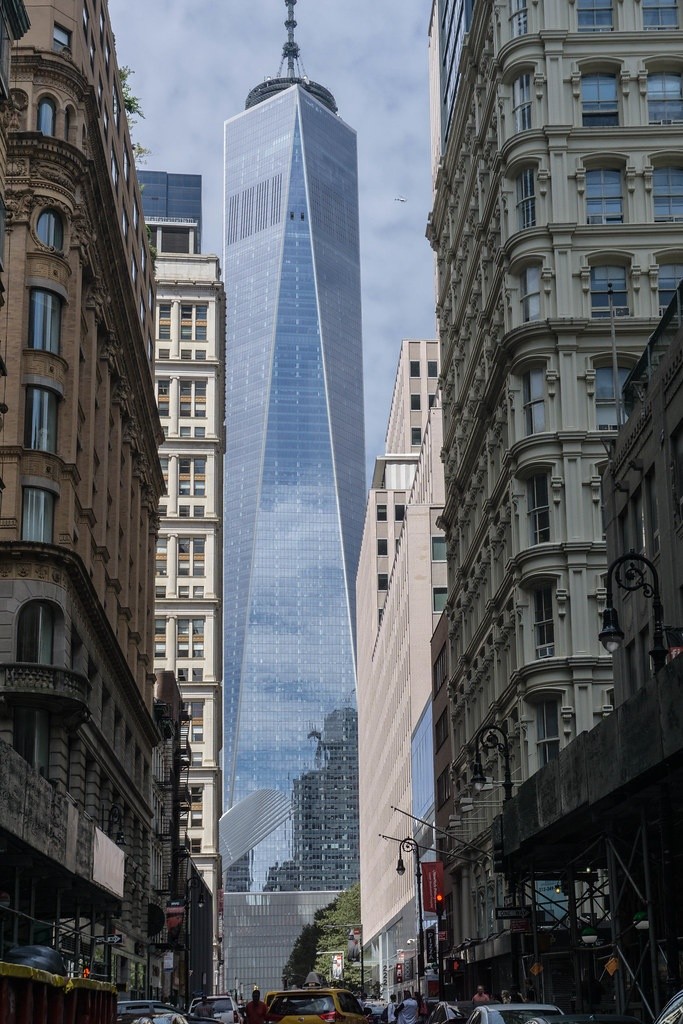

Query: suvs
[188,995,243,1024]
[112,1000,224,1024]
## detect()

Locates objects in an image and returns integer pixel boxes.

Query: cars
[266,989,372,1024]
[363,1000,386,1024]
[466,988,683,1024]
[426,1001,501,1024]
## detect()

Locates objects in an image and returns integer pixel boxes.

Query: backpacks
[381,1003,393,1023]
[419,1001,429,1016]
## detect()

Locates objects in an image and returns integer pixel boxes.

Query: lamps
[434,798,504,839]
[475,776,525,792]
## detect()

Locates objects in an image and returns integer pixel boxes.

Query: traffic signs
[94,934,122,944]
[495,907,532,919]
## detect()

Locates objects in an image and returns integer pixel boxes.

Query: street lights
[106,805,126,983]
[396,837,423,975]
[470,725,523,1005]
[323,924,363,996]
[185,876,205,1011]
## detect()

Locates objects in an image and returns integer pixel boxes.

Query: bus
[264,991,278,1007]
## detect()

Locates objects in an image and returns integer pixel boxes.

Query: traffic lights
[436,895,443,916]
[450,960,464,973]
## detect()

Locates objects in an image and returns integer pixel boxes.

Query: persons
[245,990,269,1024]
[500,979,538,1004]
[194,994,214,1018]
[472,985,490,1002]
[394,991,419,1024]
[381,994,400,1024]
[413,992,427,1024]
[359,993,368,1011]
